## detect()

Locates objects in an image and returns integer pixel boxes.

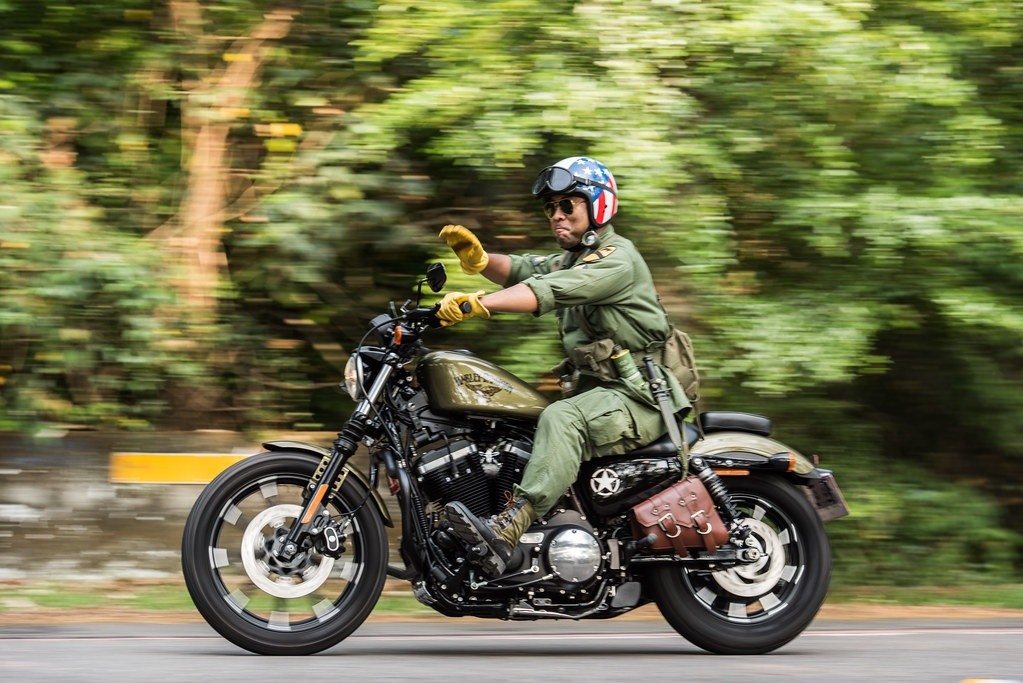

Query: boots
[444,490,536,576]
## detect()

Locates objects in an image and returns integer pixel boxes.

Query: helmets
[550,156,618,227]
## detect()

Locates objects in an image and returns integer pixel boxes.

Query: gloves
[435,290,490,326]
[439,224,489,275]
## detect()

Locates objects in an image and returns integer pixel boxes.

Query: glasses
[531,166,577,198]
[544,198,587,220]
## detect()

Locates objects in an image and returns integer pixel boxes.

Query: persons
[435,157,700,576]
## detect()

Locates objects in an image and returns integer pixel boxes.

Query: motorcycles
[180,264,849,655]
